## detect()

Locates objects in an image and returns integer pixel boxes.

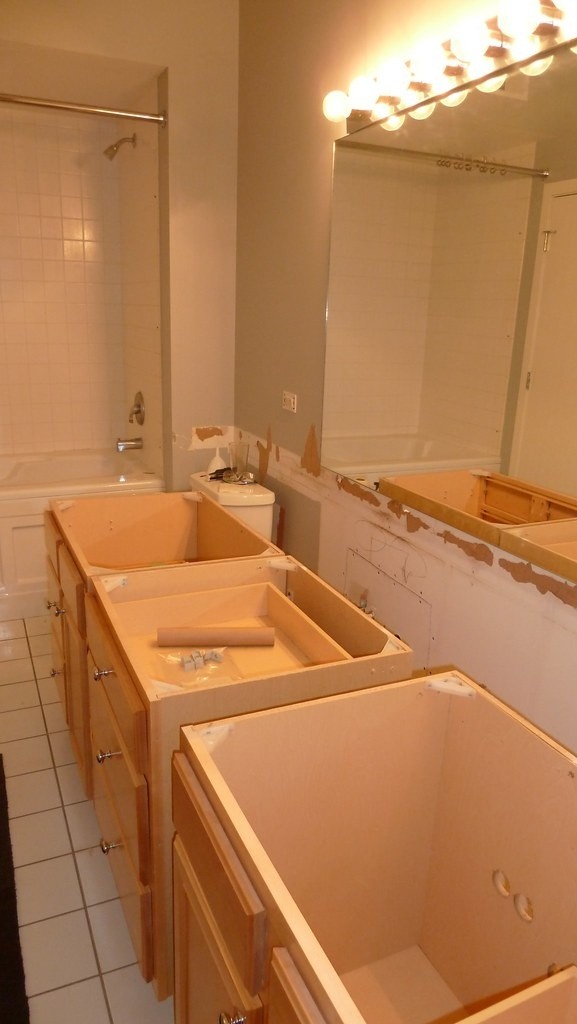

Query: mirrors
[319,38,577,586]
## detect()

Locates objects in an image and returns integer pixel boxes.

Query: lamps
[321,0,577,123]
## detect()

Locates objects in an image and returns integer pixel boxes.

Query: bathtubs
[1,447,166,621]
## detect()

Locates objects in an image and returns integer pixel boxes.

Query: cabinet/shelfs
[44,490,285,800]
[379,468,577,548]
[171,669,577,1024]
[499,520,577,583]
[82,555,414,984]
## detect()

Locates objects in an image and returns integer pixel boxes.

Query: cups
[228,442,249,474]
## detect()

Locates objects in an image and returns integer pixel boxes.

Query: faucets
[116,437,143,453]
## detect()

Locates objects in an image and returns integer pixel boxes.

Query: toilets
[189,471,276,544]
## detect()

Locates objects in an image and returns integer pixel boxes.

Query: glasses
[223,470,257,485]
